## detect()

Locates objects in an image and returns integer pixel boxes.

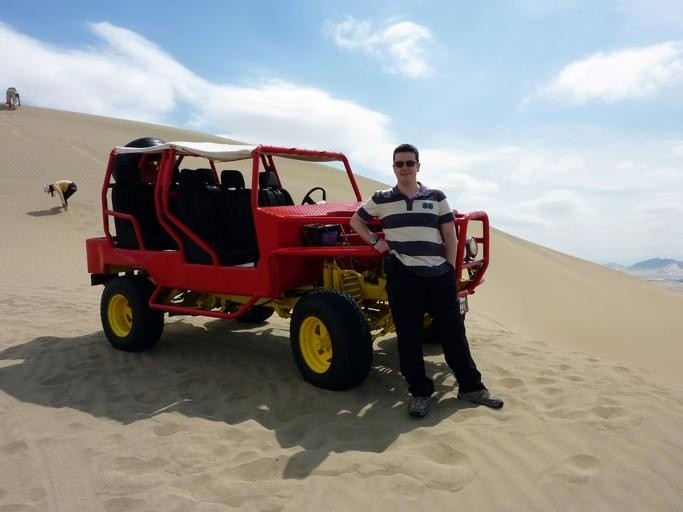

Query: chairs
[111,169,295,266]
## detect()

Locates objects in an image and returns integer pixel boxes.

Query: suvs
[85,138,491,390]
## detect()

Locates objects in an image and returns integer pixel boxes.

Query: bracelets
[367,232,380,246]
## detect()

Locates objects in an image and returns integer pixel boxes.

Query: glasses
[394,160,417,168]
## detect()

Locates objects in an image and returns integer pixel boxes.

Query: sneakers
[408,396,431,417]
[457,390,503,408]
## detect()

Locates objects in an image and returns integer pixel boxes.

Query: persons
[347,139,508,420]
[4,85,22,110]
[42,178,78,211]
